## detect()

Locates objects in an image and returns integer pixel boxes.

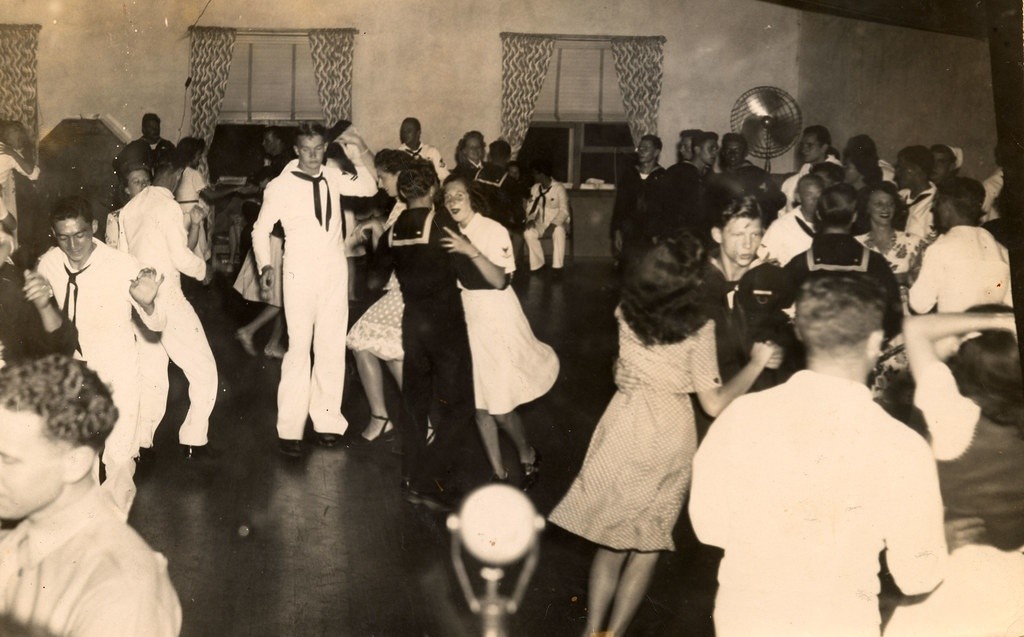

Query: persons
[547,128,1024,637]
[0,351,182,637]
[234,117,571,511]
[250,122,377,458]
[685,274,985,637]
[0,112,246,521]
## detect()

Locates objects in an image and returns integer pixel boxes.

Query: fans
[731,82,805,176]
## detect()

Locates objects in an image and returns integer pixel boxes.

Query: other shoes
[234,328,258,356]
[553,267,564,276]
[182,279,196,300]
[318,433,341,448]
[279,439,302,457]
[405,472,462,512]
[536,265,546,274]
[264,344,285,359]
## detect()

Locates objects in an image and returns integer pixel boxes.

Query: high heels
[344,415,396,449]
[423,400,458,456]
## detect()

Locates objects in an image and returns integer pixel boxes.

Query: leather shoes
[490,466,512,482]
[520,447,541,480]
[139,447,156,464]
[183,446,222,460]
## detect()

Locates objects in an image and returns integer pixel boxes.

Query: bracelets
[361,148,368,155]
[468,250,481,259]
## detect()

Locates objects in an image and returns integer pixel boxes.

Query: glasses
[635,145,654,152]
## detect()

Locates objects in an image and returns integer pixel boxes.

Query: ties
[795,216,818,242]
[291,171,332,232]
[62,264,89,356]
[406,147,422,159]
[529,185,552,223]
[905,194,931,208]
